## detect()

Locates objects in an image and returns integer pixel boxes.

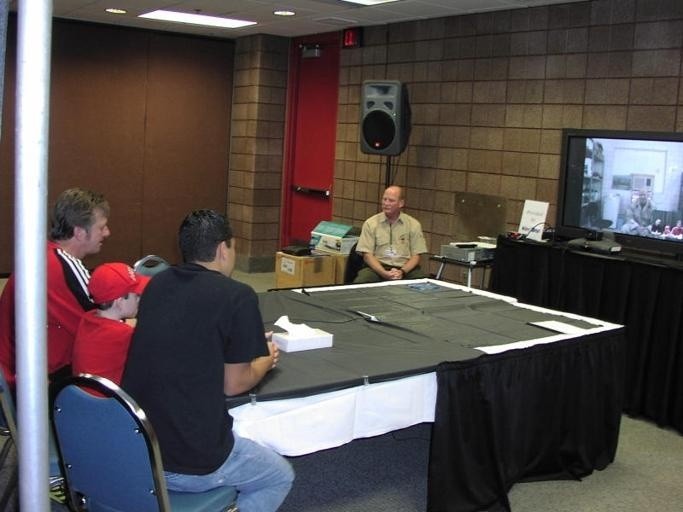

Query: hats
[87,263,152,305]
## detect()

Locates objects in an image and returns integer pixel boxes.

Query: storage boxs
[308,221,363,255]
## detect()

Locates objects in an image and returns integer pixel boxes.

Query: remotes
[456,244,478,248]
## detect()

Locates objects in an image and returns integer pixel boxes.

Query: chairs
[343,242,371,284]
[0,366,239,512]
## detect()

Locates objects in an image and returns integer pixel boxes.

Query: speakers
[359,79,413,155]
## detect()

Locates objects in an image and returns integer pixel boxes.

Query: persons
[349,185,429,284]
[69,262,153,398]
[620,190,683,240]
[120,207,295,512]
[0,186,112,504]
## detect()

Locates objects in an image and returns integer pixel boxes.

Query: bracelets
[400,268,406,278]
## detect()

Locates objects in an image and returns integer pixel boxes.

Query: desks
[428,255,495,288]
[227,234,683,511]
[228,278,626,512]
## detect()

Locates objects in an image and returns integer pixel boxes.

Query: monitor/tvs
[555,127,683,263]
